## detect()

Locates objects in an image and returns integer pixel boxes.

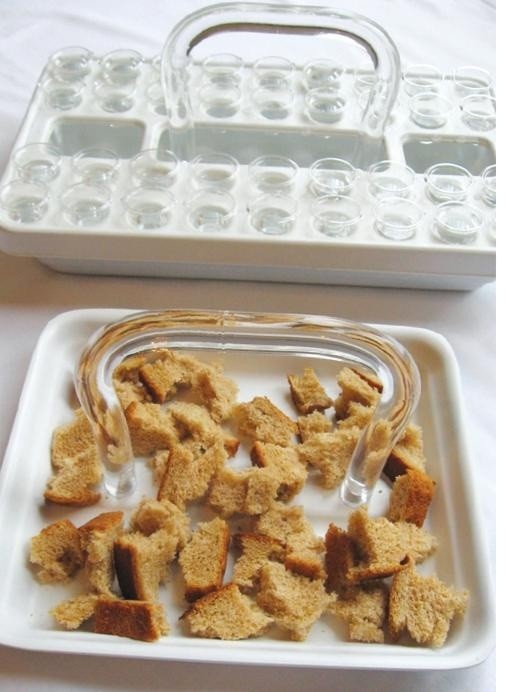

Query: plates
[0,306,500,673]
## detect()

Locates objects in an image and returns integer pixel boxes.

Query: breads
[30,342,471,648]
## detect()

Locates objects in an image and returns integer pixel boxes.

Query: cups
[366,160,416,197]
[247,194,299,237]
[253,80,295,112]
[426,162,474,200]
[306,87,346,123]
[14,143,63,179]
[147,80,171,104]
[308,155,357,194]
[302,59,345,90]
[125,187,173,228]
[373,196,425,239]
[60,183,115,228]
[435,200,484,243]
[410,92,452,126]
[461,94,494,125]
[190,151,241,192]
[98,48,142,81]
[403,63,445,95]
[96,81,135,112]
[454,67,491,98]
[354,62,375,91]
[73,145,118,186]
[2,182,54,223]
[197,80,242,111]
[484,164,495,193]
[202,53,243,73]
[254,57,292,82]
[151,53,165,71]
[246,157,300,192]
[43,81,91,114]
[130,147,177,186]
[49,48,92,78]
[310,193,365,238]
[187,193,237,232]
